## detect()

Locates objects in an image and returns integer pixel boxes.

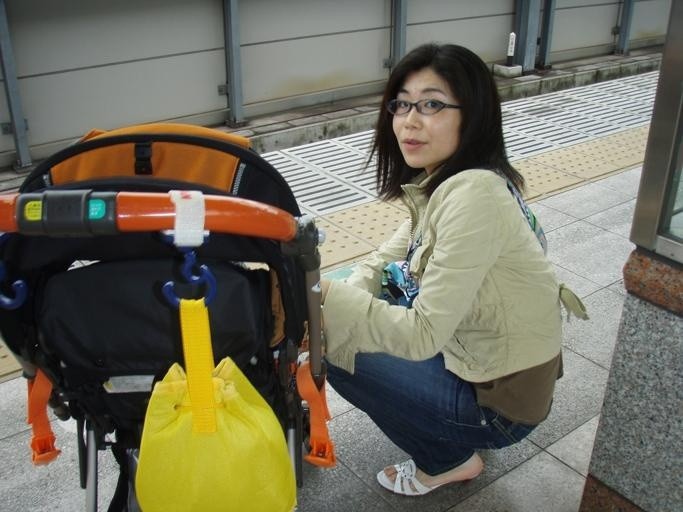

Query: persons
[320,44,588,496]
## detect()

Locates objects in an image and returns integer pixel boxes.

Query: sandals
[376,459,482,496]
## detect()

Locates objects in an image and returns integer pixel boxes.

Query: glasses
[386,100,461,115]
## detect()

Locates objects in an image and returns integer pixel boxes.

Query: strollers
[0,123,327,512]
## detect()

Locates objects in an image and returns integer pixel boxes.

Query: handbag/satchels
[135,299,296,512]
[378,261,419,309]
